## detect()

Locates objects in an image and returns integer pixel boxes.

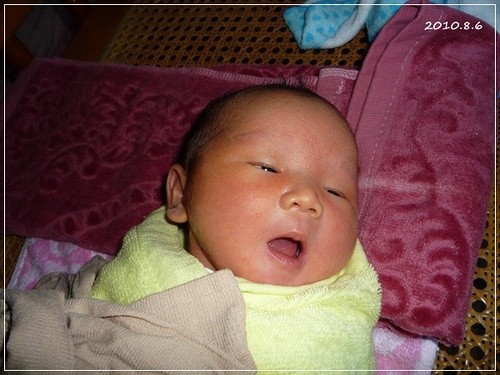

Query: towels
[0,268,257,375]
[6,236,439,375]
[90,205,384,375]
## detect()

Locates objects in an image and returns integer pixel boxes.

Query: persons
[91,80,383,375]
